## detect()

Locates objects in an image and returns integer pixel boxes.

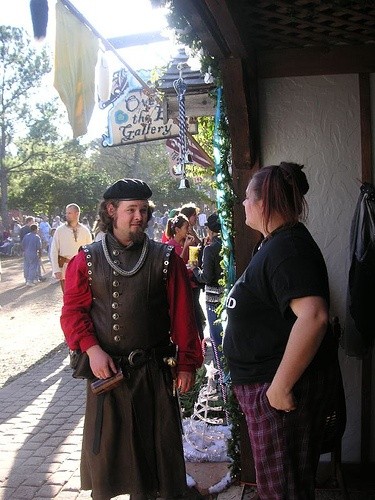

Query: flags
[54,1,100,138]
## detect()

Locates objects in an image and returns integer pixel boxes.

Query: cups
[90,367,131,396]
[189,246,200,265]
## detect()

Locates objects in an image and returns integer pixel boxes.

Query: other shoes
[26,282,35,286]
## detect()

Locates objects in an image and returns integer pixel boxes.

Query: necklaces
[100,233,149,277]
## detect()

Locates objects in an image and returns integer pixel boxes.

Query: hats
[103,178,151,200]
[204,214,220,232]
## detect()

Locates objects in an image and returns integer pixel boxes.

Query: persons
[223,160,331,500]
[50,203,93,293]
[60,178,205,500]
[147,201,224,369]
[0,214,63,287]
[82,215,106,242]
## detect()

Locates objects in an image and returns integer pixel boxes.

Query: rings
[285,409,291,413]
